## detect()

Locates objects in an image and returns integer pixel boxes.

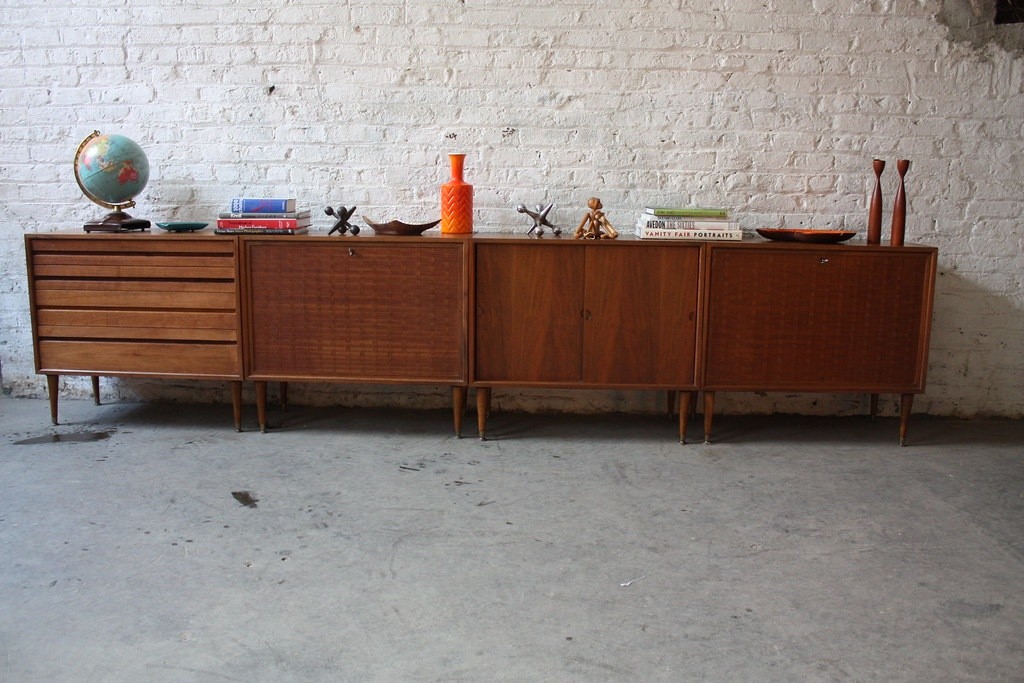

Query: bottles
[441,153,474,233]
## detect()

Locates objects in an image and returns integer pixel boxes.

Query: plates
[156,222,209,233]
[362,215,442,236]
[755,228,856,243]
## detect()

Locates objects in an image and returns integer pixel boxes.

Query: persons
[573,197,618,240]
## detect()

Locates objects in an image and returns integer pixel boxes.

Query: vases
[440,153,473,234]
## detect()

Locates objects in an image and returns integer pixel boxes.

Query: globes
[72,130,152,233]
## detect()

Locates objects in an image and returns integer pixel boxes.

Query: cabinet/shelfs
[690,236,938,446]
[24,230,243,433]
[240,230,477,440]
[468,232,699,445]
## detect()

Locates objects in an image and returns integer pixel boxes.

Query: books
[213,198,313,236]
[634,206,743,241]
